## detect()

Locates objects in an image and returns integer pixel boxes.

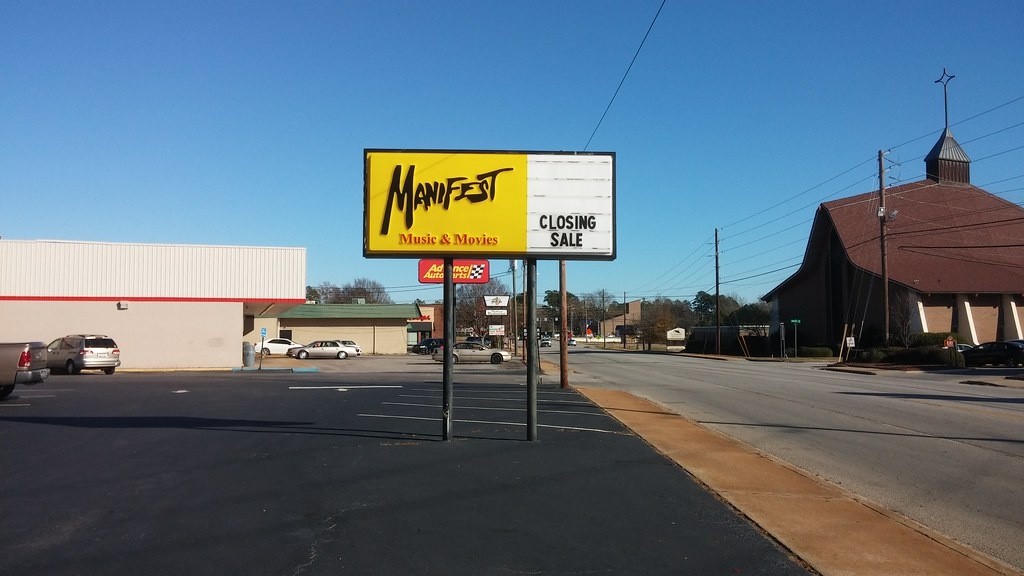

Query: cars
[568,340,577,346]
[942,339,1024,368]
[334,339,361,356]
[432,342,512,364]
[544,335,551,341]
[254,338,304,356]
[466,337,490,345]
[286,341,358,360]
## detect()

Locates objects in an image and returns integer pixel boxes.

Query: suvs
[541,339,552,347]
[412,338,443,355]
[46,334,121,376]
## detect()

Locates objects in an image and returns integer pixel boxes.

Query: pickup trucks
[0,341,52,397]
